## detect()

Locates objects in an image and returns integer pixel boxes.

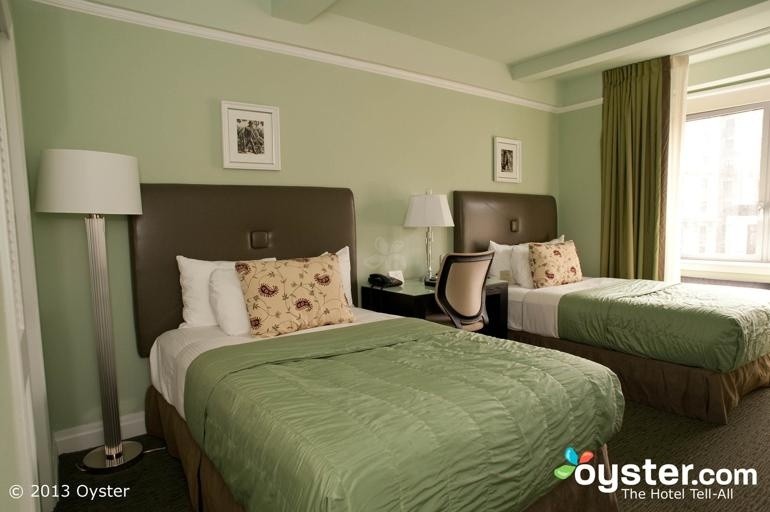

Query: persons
[503,152,511,171]
[242,121,258,154]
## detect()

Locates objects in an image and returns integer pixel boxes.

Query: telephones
[368,273,403,288]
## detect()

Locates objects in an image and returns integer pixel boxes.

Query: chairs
[426,251,495,331]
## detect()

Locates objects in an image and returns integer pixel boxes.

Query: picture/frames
[221,101,281,171]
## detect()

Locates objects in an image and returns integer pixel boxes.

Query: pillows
[176,246,356,338]
[493,137,521,183]
[488,235,582,288]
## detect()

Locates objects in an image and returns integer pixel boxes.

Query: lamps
[404,191,455,282]
[34,149,142,474]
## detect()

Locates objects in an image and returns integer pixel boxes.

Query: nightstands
[361,277,508,339]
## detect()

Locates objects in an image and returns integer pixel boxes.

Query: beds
[129,184,625,512]
[454,191,770,425]
